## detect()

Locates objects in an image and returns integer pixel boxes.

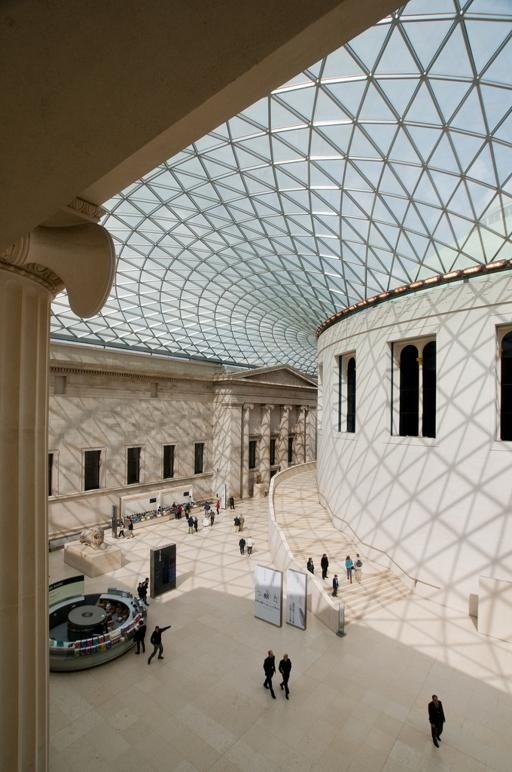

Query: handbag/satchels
[351,566,355,569]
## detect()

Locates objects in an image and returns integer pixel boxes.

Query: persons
[428,695,445,748]
[158,500,220,534]
[239,536,253,554]
[137,578,150,606]
[263,649,292,700]
[307,553,363,597]
[229,496,235,509]
[118,519,134,539]
[234,514,244,532]
[133,620,172,665]
[105,601,111,610]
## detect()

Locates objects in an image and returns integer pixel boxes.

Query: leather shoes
[271,694,276,699]
[286,695,289,699]
[433,736,441,748]
[263,683,269,689]
[280,684,284,690]
[135,652,140,654]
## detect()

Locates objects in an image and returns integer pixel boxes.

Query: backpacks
[356,560,362,567]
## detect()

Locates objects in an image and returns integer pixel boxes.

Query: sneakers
[148,658,151,664]
[158,656,164,659]
[143,649,145,652]
